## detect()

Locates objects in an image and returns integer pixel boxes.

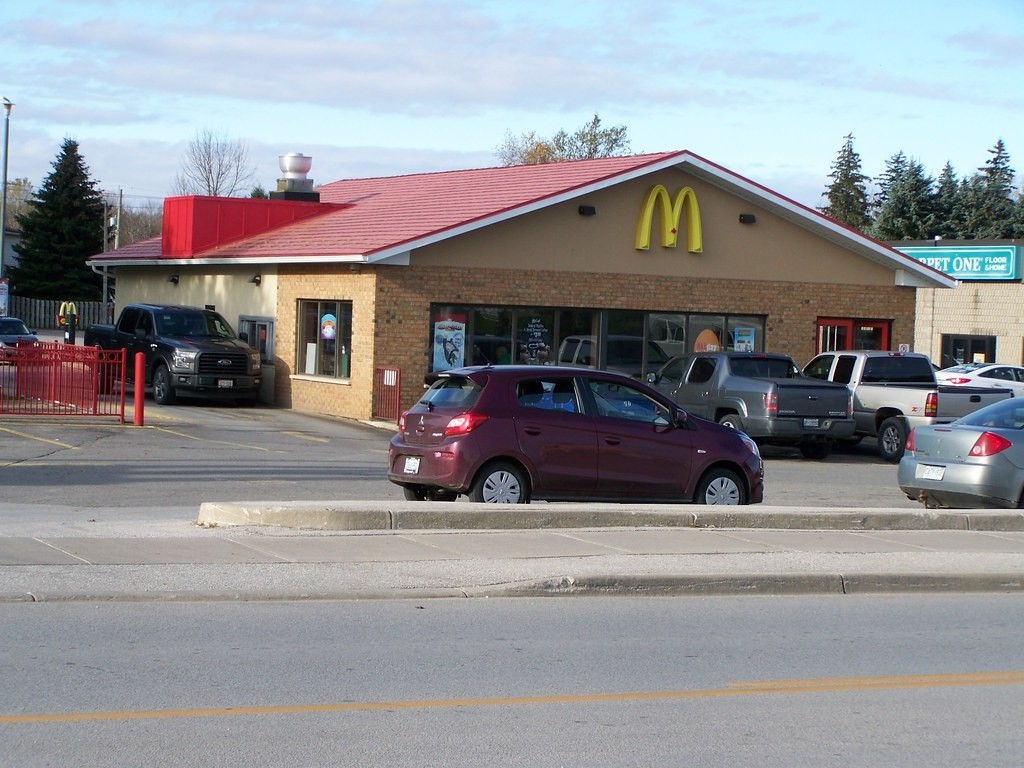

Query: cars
[647,350,858,460]
[898,394,1024,510]
[933,362,1024,396]
[0,317,42,365]
[386,360,765,506]
[557,334,675,380]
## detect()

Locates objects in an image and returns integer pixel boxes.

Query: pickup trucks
[799,347,1015,465]
[84,303,263,408]
